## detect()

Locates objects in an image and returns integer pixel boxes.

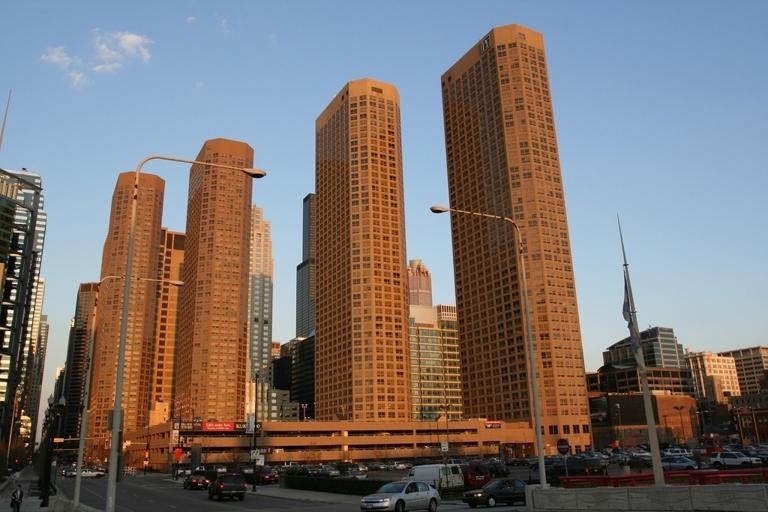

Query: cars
[209,473,248,502]
[359,480,442,511]
[590,408,607,422]
[463,477,527,508]
[183,475,210,491]
[254,461,414,484]
[62,461,105,480]
[465,461,492,486]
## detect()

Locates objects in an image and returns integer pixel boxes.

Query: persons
[9,471,16,489]
[504,482,511,488]
[12,484,24,512]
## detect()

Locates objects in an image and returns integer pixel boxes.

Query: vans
[402,464,465,488]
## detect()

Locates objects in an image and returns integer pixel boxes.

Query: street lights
[73,275,184,511]
[159,398,181,481]
[252,368,262,491]
[430,205,548,488]
[105,155,268,512]
[672,405,686,442]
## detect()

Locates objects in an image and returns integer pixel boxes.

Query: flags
[622,270,646,383]
